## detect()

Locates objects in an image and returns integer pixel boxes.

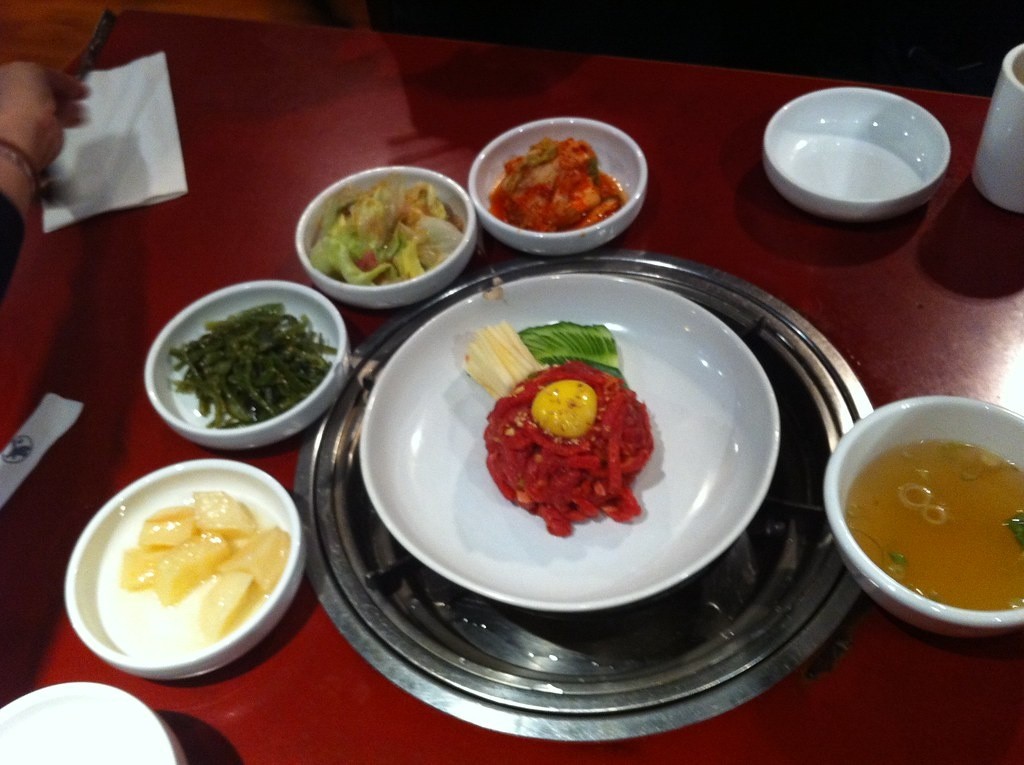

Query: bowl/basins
[64,457,303,685]
[144,279,352,452]
[0,680,190,765]
[466,117,650,256]
[295,164,479,310]
[763,87,955,222]
[823,393,1022,639]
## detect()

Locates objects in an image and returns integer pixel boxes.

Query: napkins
[22,53,188,229]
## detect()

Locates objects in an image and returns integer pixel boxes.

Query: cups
[968,43,1023,213]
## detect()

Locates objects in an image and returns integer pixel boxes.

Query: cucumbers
[522,320,624,382]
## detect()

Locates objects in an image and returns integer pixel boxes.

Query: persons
[0,57,90,306]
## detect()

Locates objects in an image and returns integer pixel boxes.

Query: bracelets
[0,135,41,192]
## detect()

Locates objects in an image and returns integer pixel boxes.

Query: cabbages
[489,134,622,230]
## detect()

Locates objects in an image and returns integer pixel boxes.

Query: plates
[358,273,779,610]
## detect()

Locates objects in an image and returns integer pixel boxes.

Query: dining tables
[2,9,1024,764]
